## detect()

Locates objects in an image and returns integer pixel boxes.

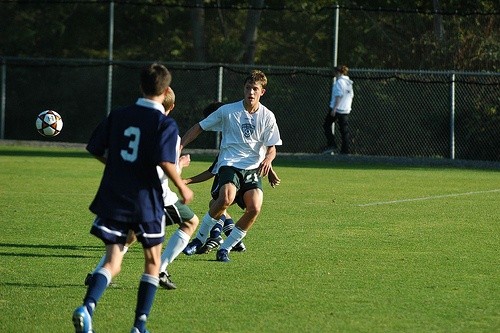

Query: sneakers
[216,249,230,261]
[182,240,199,255]
[230,241,246,252]
[85,273,93,286]
[159,271,177,289]
[201,235,224,254]
[72,306,94,333]
[130,319,149,333]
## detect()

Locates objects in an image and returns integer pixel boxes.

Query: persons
[85,86,199,289]
[181,71,282,262]
[72,64,194,333]
[324,65,354,154]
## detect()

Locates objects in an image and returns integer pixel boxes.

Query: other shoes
[324,146,337,153]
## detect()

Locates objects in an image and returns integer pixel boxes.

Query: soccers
[36,109,64,137]
